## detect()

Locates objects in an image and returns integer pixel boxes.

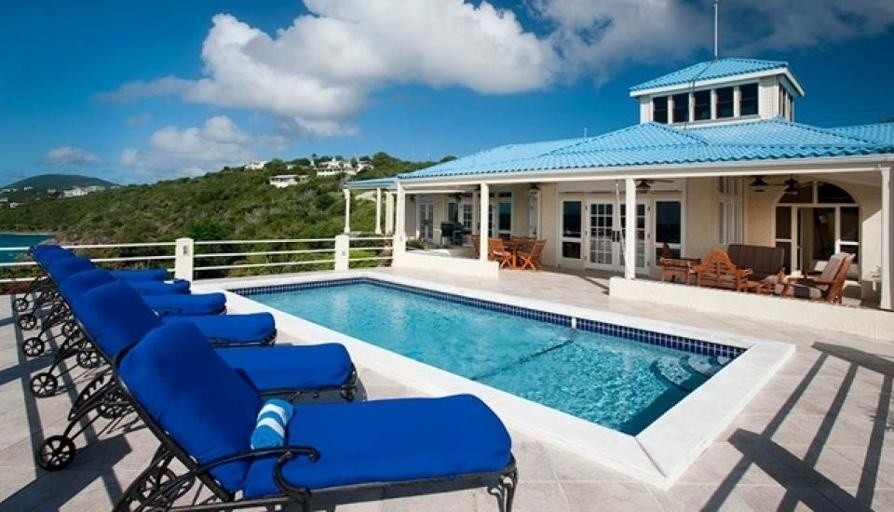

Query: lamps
[455,193,460,201]
[409,194,415,202]
[635,180,650,193]
[528,183,540,195]
[748,176,802,195]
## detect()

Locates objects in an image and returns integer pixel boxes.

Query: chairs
[469,233,547,271]
[100,319,519,511]
[30,279,365,472]
[660,241,857,304]
[11,245,282,401]
